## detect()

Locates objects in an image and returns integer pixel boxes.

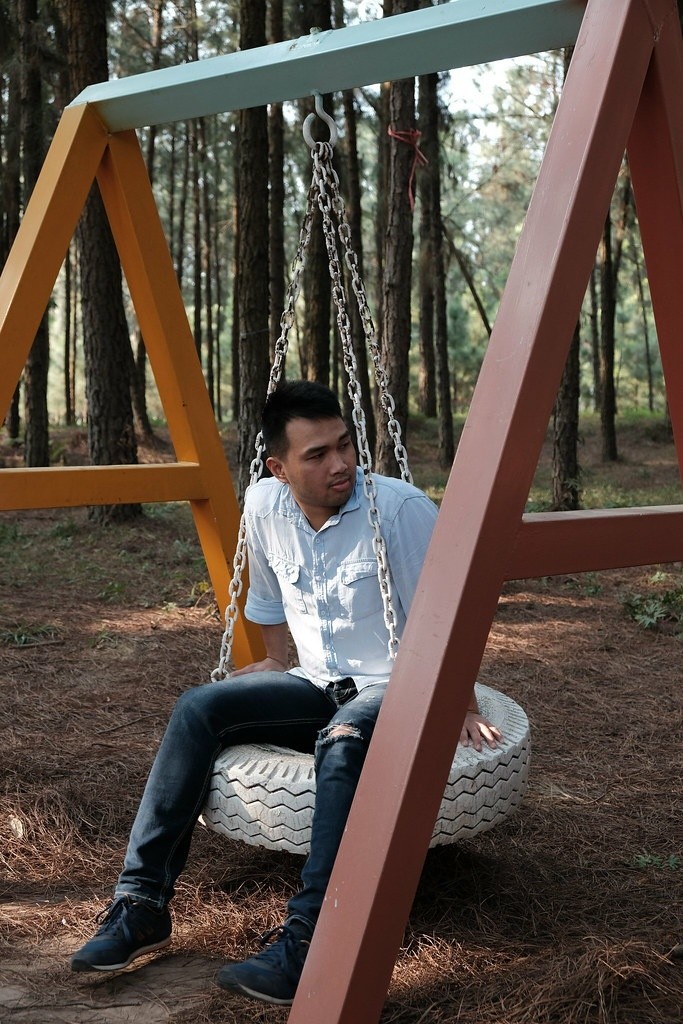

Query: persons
[68,379,504,1003]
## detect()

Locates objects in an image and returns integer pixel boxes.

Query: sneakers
[217,926,312,1004]
[71,894,173,972]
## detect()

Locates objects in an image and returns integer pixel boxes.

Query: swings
[199,137,534,857]
